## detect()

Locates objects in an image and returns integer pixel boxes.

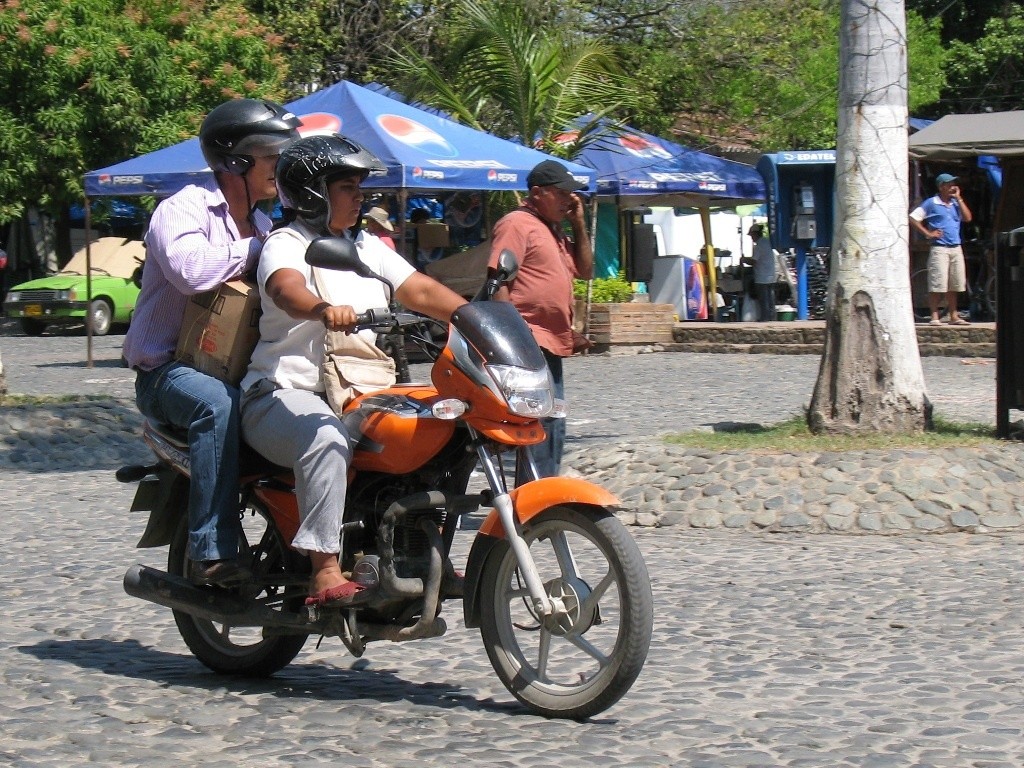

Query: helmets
[200,99,304,175]
[276,133,388,239]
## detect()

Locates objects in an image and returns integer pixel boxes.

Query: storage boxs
[174,274,263,384]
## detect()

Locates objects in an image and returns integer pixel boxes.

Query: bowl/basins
[776,311,797,320]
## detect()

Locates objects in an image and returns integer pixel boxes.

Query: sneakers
[190,561,252,585]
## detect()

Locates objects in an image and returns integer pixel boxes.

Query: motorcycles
[115,235,654,720]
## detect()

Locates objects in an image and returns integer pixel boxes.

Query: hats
[362,207,394,231]
[527,160,591,192]
[935,173,960,186]
[746,223,763,234]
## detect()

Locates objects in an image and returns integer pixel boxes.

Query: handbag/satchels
[323,329,396,419]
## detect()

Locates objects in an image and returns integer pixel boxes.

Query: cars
[5,235,147,335]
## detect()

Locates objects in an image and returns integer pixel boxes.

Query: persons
[485,160,594,491]
[242,135,473,608]
[361,207,400,271]
[124,101,302,587]
[908,174,973,326]
[739,224,780,323]
[409,207,438,270]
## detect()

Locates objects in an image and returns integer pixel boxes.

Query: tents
[908,110,1024,158]
[504,112,770,320]
[79,78,597,368]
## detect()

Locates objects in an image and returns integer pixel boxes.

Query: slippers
[950,319,970,326]
[930,320,942,326]
[304,581,367,609]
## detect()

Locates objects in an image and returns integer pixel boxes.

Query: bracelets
[956,199,964,202]
